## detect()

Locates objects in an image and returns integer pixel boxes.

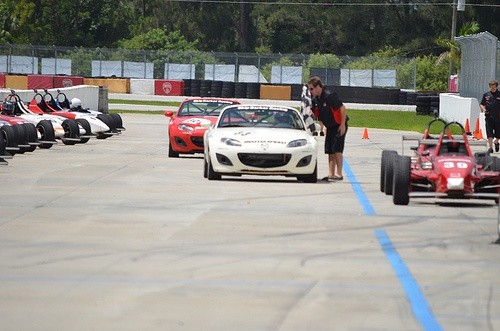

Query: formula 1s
[0,88,126,165]
[379,118,500,206]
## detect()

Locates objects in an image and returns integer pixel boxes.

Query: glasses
[310,86,314,91]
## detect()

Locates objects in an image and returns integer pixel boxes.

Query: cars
[163,96,242,158]
[200,104,319,184]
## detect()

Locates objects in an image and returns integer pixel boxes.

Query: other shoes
[495,142,499,152]
[328,175,343,182]
[320,176,331,181]
[488,148,493,153]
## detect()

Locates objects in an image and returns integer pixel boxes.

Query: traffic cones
[362,128,370,140]
[464,119,472,135]
[473,118,479,138]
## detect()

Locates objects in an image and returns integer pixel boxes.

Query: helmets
[70,98,81,109]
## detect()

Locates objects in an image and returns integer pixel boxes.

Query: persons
[480,80,500,153]
[308,76,350,181]
